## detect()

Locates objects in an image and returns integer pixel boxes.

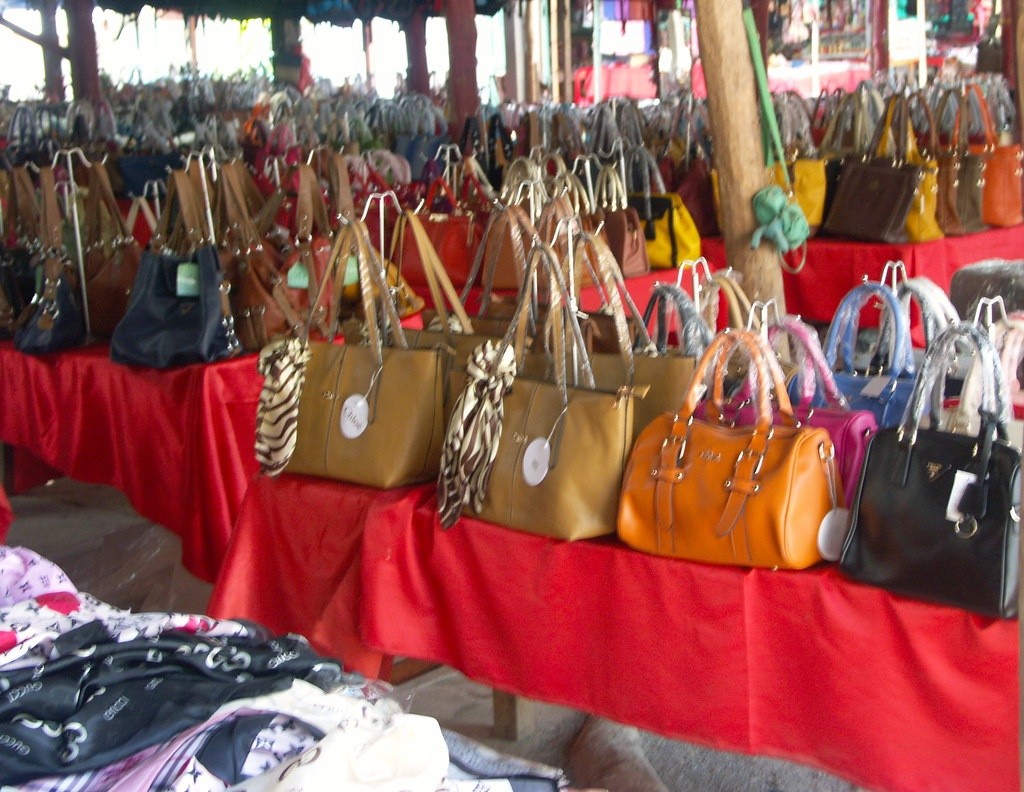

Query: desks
[0,62,1024,792]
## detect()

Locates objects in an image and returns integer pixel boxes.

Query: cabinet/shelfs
[768,0,924,61]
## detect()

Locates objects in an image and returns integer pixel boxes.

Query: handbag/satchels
[0,0,1023,620]
